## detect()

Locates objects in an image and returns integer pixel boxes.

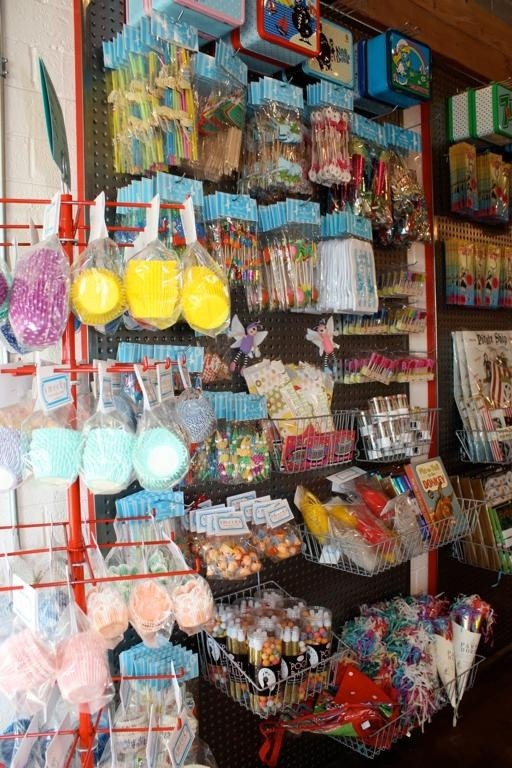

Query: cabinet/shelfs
[76,0,493,768]
[0,195,204,768]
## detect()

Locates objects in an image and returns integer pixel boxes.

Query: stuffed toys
[227,313,269,379]
[303,316,343,374]
